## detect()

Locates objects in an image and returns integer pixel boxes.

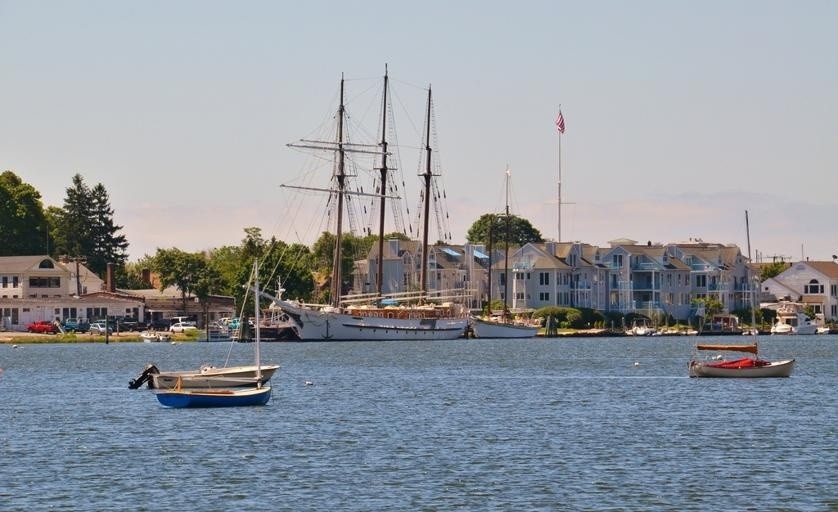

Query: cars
[25,316,253,335]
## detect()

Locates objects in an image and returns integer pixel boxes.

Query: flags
[556,112,564,134]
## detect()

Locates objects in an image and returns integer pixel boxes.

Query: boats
[147,365,280,389]
[771,313,817,334]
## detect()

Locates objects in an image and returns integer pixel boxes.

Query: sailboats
[155,257,272,409]
[687,209,794,377]
